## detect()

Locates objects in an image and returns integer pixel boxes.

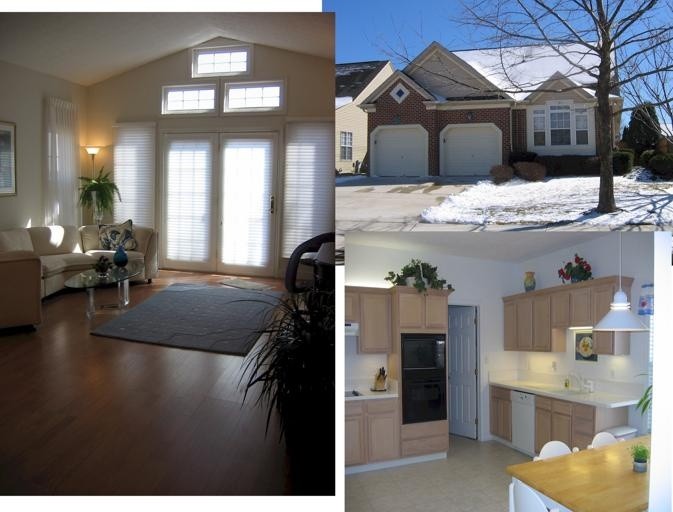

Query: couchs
[0,222,159,300]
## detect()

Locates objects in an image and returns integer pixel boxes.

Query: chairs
[533,440,579,462]
[507,482,561,512]
[0,250,42,333]
[587,431,624,449]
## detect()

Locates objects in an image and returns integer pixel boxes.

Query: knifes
[376,367,387,382]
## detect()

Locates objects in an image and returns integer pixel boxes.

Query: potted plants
[77,166,122,225]
[630,442,649,472]
[384,258,453,294]
[91,255,113,278]
[214,282,335,495]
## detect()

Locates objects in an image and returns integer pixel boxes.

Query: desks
[506,434,651,512]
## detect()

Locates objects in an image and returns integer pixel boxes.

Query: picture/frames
[572,330,599,365]
[0,121,17,197]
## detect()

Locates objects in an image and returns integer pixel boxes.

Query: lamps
[83,145,102,228]
[593,232,650,332]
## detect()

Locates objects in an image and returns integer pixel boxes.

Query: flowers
[558,254,591,281]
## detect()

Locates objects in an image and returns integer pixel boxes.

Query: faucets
[569,371,583,393]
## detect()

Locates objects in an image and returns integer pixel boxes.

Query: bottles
[564,379,568,387]
[637,281,654,317]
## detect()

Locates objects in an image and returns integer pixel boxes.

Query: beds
[64,268,141,320]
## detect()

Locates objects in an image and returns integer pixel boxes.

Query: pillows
[98,219,137,251]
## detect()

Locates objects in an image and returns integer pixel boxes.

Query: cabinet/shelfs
[489,387,512,441]
[357,293,390,355]
[346,400,365,467]
[535,395,572,451]
[365,398,401,464]
[345,291,357,322]
[501,275,634,354]
[399,289,448,328]
[571,403,628,449]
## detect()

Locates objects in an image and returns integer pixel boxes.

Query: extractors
[344,322,359,336]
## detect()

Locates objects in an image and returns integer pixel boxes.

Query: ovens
[401,338,446,424]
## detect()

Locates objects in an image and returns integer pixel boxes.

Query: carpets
[90,281,284,357]
[220,278,277,291]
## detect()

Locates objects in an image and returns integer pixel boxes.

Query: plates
[578,336,593,358]
